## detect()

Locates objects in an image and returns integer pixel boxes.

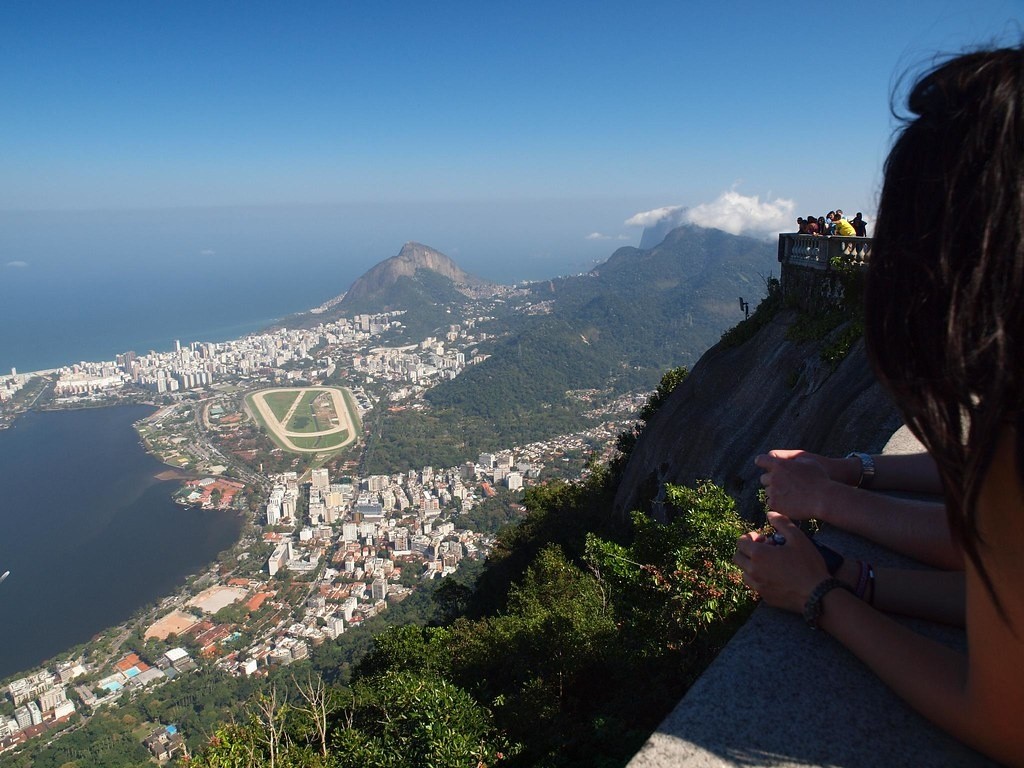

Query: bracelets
[856,561,868,600]
[866,560,877,606]
[803,578,852,631]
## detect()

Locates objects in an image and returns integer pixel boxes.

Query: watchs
[845,451,875,492]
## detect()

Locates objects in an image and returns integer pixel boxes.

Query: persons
[754,445,969,571]
[797,210,867,256]
[732,46,1024,768]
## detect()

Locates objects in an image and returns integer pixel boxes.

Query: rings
[765,497,769,505]
